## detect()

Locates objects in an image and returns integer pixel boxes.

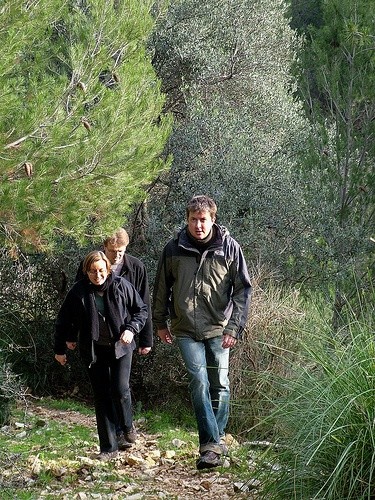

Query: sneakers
[196,450,222,470]
[219,436,228,454]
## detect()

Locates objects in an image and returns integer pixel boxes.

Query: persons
[54,226,152,460]
[152,195,253,468]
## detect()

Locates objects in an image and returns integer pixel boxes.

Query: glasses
[87,269,107,275]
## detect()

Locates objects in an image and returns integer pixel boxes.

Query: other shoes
[123,422,137,443]
[117,434,132,449]
[93,450,118,462]
